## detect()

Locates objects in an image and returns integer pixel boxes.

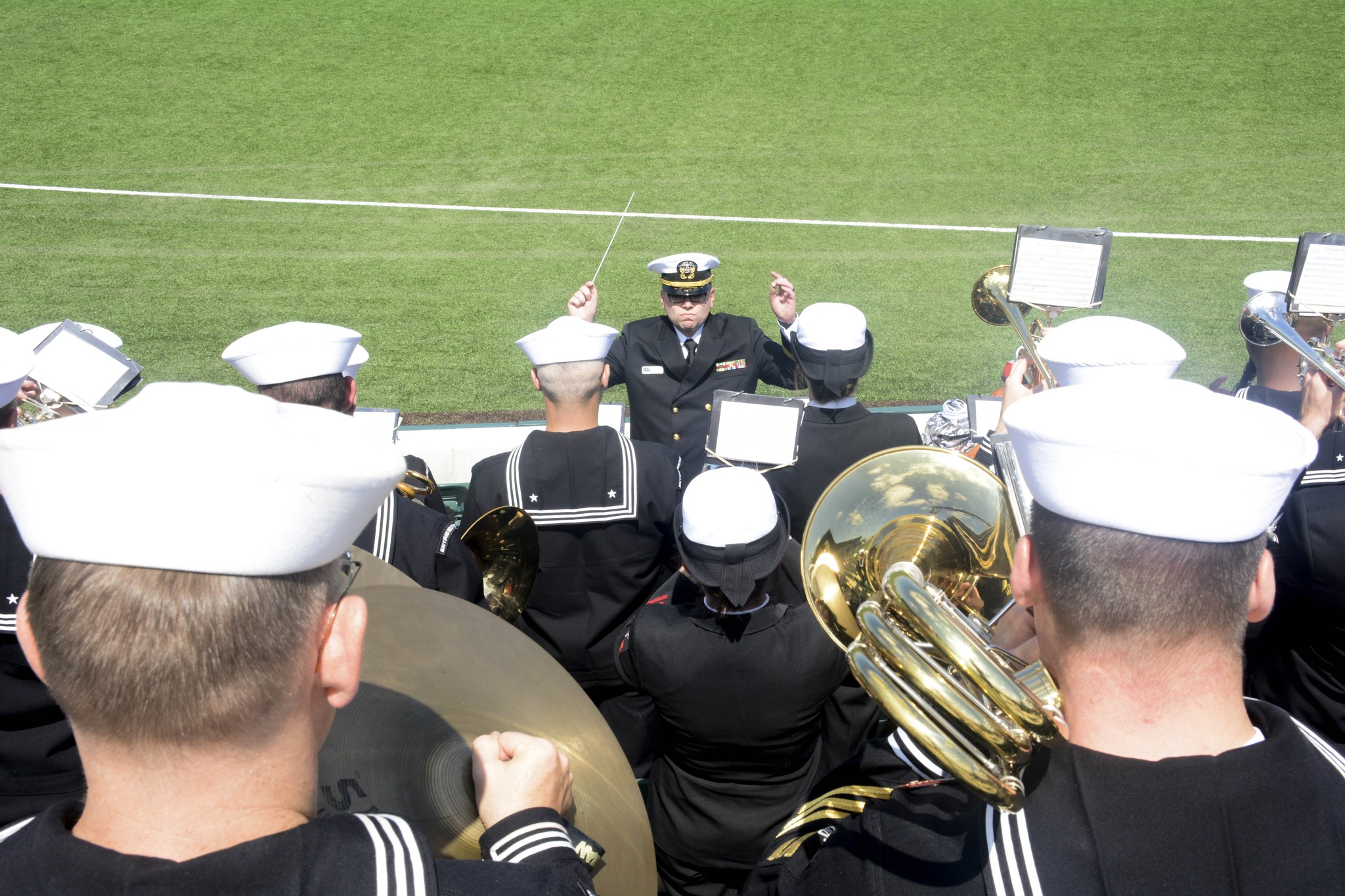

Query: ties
[683,337,698,368]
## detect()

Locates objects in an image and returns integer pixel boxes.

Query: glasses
[664,291,708,304]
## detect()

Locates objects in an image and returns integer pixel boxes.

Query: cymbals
[321,545,657,896]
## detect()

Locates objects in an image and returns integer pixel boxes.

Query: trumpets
[1239,290,1345,424]
[971,264,1058,393]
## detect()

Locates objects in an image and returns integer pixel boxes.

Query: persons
[568,252,808,480]
[0,270,1345,896]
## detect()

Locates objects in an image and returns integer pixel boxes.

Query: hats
[789,303,875,396]
[1245,270,1323,316]
[340,343,370,381]
[647,253,720,297]
[0,381,406,579]
[219,321,361,387]
[1002,383,1319,543]
[15,320,124,377]
[0,325,35,410]
[672,462,791,604]
[1037,317,1188,387]
[518,314,619,368]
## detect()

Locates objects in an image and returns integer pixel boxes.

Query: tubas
[802,445,1063,813]
